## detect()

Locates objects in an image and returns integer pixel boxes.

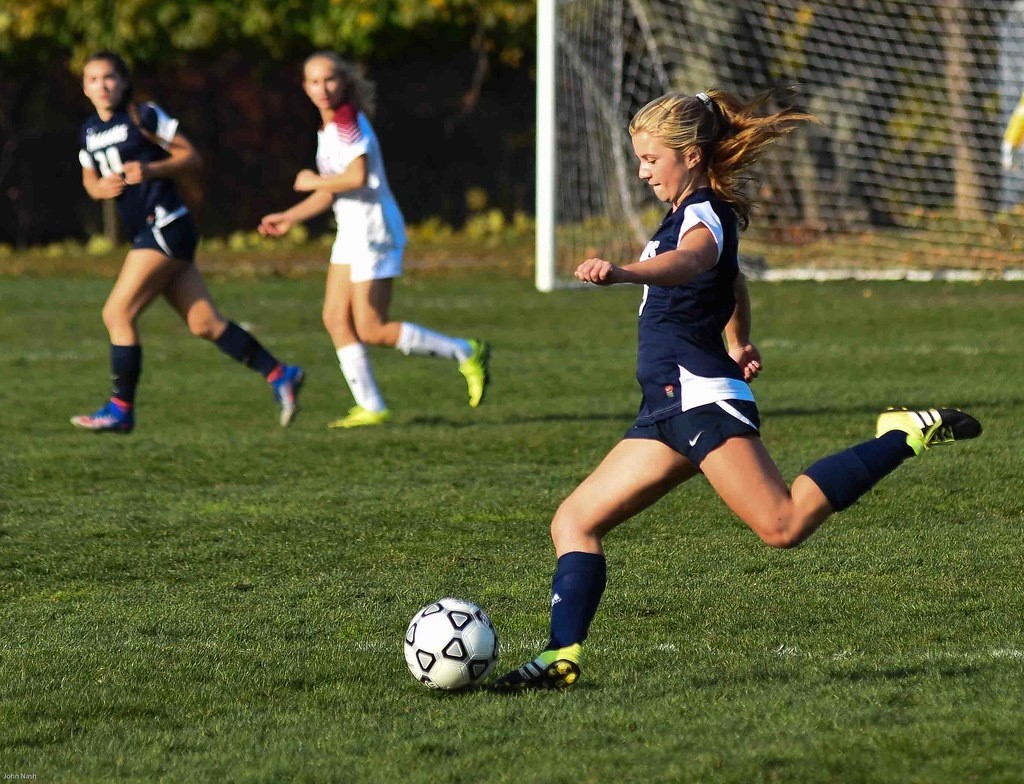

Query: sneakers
[458,339,490,407]
[329,405,394,427]
[268,363,304,428]
[489,642,583,690]
[875,406,982,458]
[69,396,133,431]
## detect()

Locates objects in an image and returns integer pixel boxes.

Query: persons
[70,48,306,434]
[481,88,983,696]
[257,52,492,429]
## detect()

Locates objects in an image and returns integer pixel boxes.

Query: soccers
[402,597,500,692]
